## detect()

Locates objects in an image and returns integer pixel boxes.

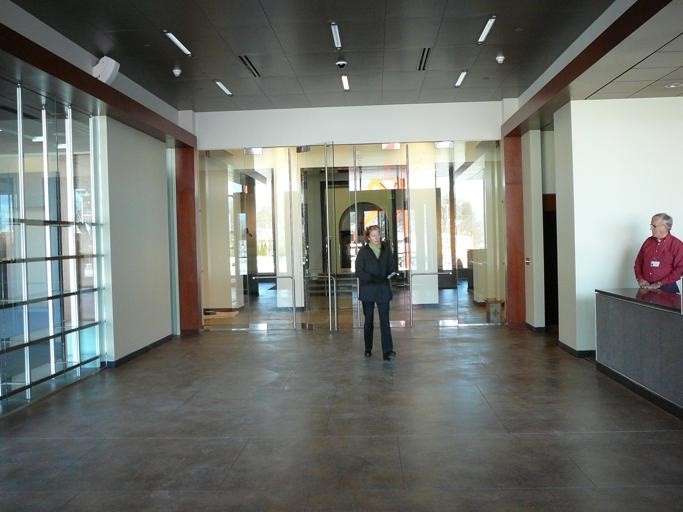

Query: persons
[354,225,398,361]
[632,211,683,293]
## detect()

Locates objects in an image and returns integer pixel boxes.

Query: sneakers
[364,351,372,357]
[383,350,396,361]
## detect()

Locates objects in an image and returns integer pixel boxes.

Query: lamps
[169,64,184,79]
[334,55,348,69]
[495,55,508,65]
